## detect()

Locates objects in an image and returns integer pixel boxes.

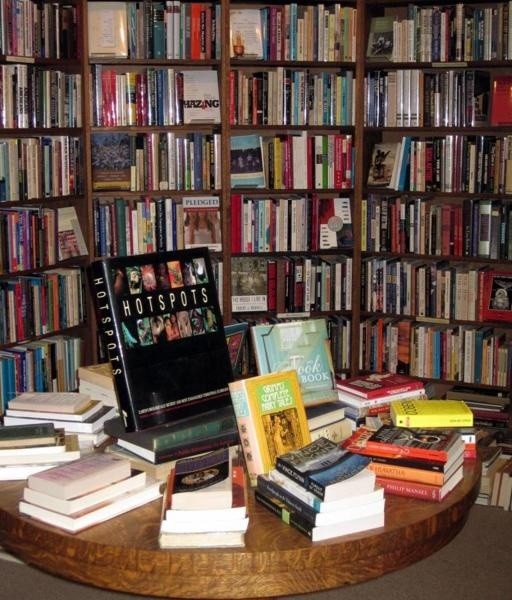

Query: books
[1,67,84,130]
[228,369,312,485]
[88,66,222,128]
[233,193,353,250]
[235,319,350,373]
[2,1,79,70]
[92,131,220,194]
[363,198,512,261]
[0,337,83,411]
[254,320,339,409]
[365,68,511,130]
[18,416,251,552]
[368,136,511,196]
[1,205,87,274]
[85,247,234,433]
[232,258,351,315]
[362,258,511,325]
[89,198,222,255]
[338,369,511,512]
[1,134,86,203]
[254,437,386,541]
[229,2,358,63]
[0,364,120,483]
[367,3,512,66]
[85,2,223,61]
[229,132,355,190]
[355,318,511,386]
[229,69,357,127]
[1,268,85,342]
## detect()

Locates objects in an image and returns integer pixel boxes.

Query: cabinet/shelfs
[0,0,512,392]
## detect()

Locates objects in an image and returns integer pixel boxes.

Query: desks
[0,437,483,600]
